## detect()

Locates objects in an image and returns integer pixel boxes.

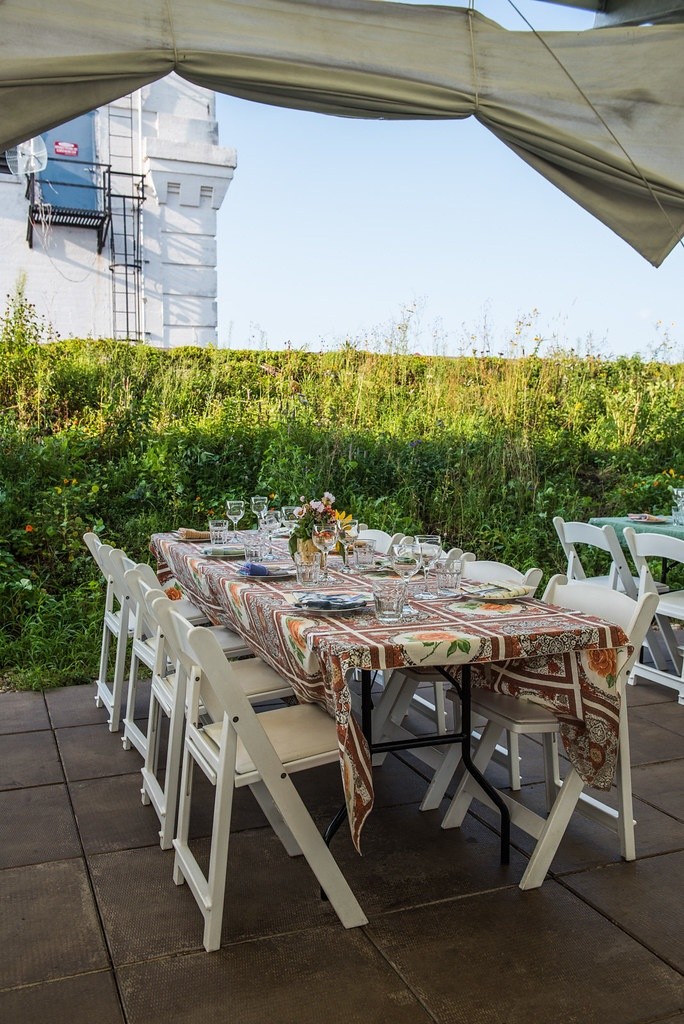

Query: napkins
[174,527,221,541]
[282,590,370,611]
[626,513,668,522]
[236,562,288,576]
[457,578,537,599]
[200,545,247,555]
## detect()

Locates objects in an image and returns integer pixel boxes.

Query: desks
[146,524,631,905]
[587,513,684,664]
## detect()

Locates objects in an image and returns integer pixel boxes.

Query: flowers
[288,490,355,560]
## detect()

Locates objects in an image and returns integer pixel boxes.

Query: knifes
[443,587,500,596]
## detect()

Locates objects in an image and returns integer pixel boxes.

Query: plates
[634,520,667,522]
[206,554,245,558]
[302,606,362,616]
[174,538,211,542]
[465,594,533,604]
[240,574,293,581]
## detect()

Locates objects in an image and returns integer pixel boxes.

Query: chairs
[82,515,684,954]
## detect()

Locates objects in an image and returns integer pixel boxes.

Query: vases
[297,534,328,569]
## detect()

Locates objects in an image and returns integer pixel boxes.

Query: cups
[672,505,684,527]
[209,519,228,547]
[373,579,405,625]
[672,488,684,506]
[294,551,322,587]
[435,559,463,597]
[353,539,376,572]
[243,533,265,564]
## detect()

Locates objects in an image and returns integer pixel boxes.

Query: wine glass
[251,496,268,535]
[392,544,423,617]
[280,506,298,559]
[337,518,359,575]
[312,524,338,582]
[412,534,442,600]
[258,511,281,562]
[227,500,245,543]
